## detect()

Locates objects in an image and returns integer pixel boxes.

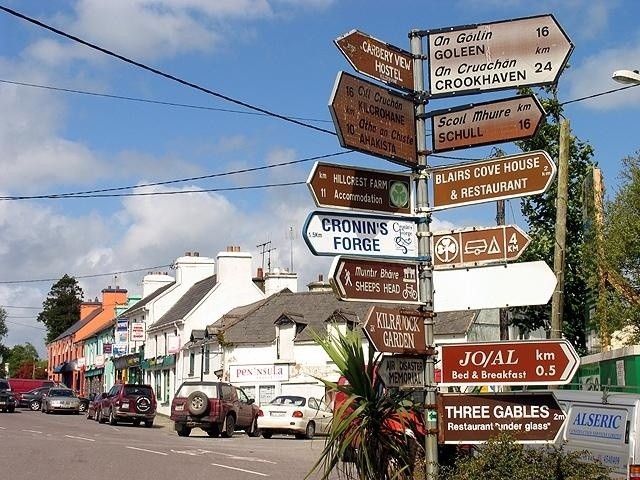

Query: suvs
[171,381,261,439]
[97,385,160,425]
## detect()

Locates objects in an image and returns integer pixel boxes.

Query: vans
[331,361,475,462]
[470,389,640,480]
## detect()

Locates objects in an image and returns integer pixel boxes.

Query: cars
[0,379,107,421]
[256,393,335,440]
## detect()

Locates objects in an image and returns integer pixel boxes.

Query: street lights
[612,70,640,85]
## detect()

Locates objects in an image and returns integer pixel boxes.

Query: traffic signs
[439,338,581,387]
[326,255,420,305]
[331,28,414,95]
[437,392,569,446]
[362,304,426,355]
[374,355,425,388]
[431,223,533,271]
[430,93,548,154]
[432,150,558,213]
[327,70,418,168]
[427,13,576,100]
[306,161,415,217]
[301,211,419,260]
